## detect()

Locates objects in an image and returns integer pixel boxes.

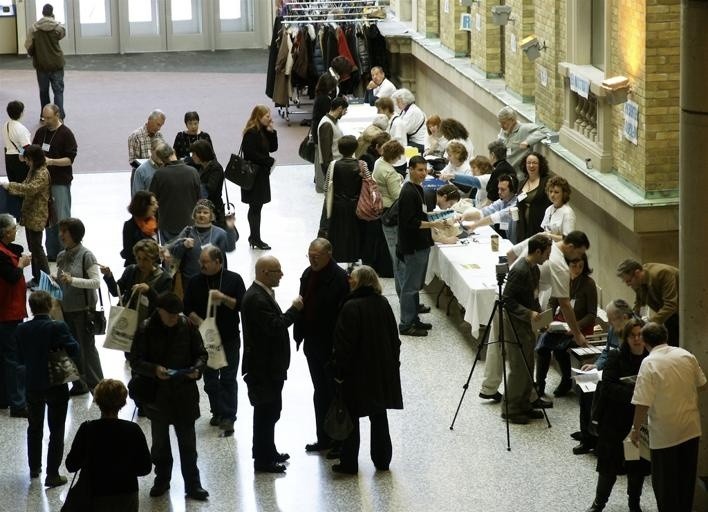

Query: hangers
[277,1,381,30]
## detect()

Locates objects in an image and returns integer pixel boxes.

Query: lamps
[460,0,478,9]
[490,4,514,27]
[521,34,545,61]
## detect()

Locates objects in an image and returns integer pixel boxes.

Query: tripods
[448,282,552,451]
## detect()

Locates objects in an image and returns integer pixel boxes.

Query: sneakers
[400,304,432,336]
[306,439,358,474]
[481,379,573,423]
[628,498,641,510]
[44,473,68,485]
[9,406,29,418]
[27,280,39,291]
[587,501,605,512]
[572,431,599,456]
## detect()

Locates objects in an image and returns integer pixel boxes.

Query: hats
[195,199,216,212]
[157,292,185,314]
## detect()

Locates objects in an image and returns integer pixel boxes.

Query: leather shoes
[255,453,290,473]
[209,414,235,438]
[186,485,209,499]
[149,482,170,496]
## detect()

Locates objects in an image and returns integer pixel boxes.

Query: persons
[242,105,279,249]
[2,214,100,486]
[130,292,209,498]
[313,56,459,335]
[0,101,78,291]
[438,107,574,241]
[25,3,67,125]
[572,260,708,512]
[96,191,246,436]
[60,380,152,512]
[479,232,597,423]
[241,256,304,472]
[127,111,224,246]
[294,239,404,474]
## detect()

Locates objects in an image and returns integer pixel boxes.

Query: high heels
[247,236,272,251]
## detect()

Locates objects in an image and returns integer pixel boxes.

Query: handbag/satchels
[224,153,261,189]
[197,316,230,370]
[51,347,79,385]
[431,218,457,244]
[60,489,91,511]
[356,179,384,221]
[104,306,139,353]
[45,197,60,230]
[325,399,354,440]
[224,211,239,243]
[86,310,106,334]
[299,136,316,164]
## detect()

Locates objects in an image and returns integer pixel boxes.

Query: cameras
[494,256,510,280]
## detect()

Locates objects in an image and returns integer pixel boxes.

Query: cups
[491,234,499,251]
[21,251,32,257]
